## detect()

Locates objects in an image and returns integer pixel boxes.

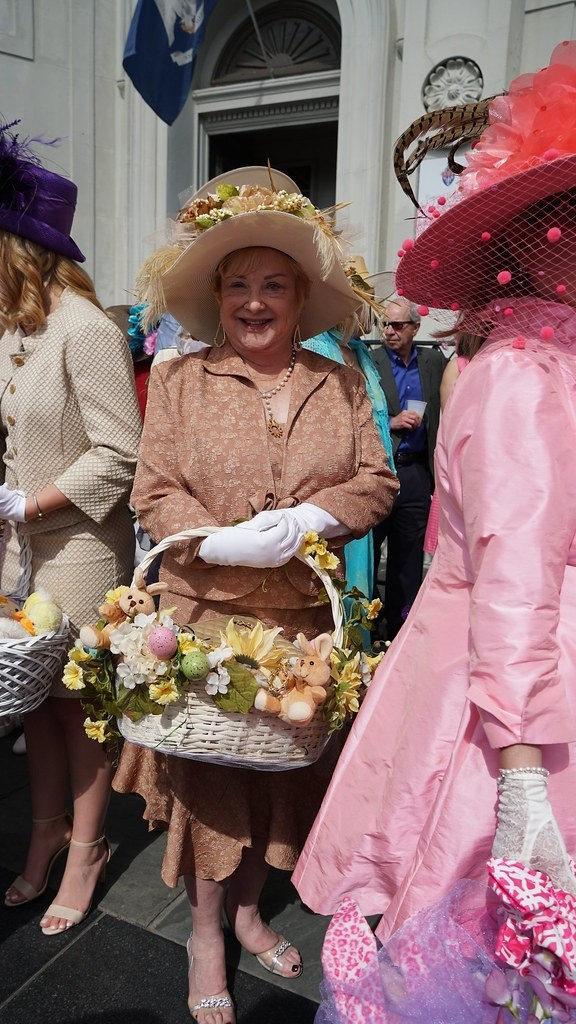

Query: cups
[407,400,427,427]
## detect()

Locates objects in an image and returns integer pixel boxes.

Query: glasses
[384,319,413,331]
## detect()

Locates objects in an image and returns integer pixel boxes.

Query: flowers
[422,59,484,114]
[61,530,391,744]
[177,181,344,239]
[128,302,159,355]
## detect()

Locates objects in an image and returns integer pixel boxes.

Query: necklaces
[263,346,295,437]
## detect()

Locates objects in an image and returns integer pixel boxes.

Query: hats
[1,119,87,262]
[142,165,387,346]
[343,255,372,286]
[396,41,576,354]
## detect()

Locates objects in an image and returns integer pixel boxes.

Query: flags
[123,0,215,127]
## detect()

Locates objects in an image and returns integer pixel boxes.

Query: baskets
[1,520,69,715]
[116,525,343,771]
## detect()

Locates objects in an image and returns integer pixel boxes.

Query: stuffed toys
[0,590,63,639]
[78,568,169,648]
[255,634,333,727]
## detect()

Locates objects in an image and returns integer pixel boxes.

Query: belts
[395,452,425,465]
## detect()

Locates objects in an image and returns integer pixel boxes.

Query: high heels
[4,811,72,906]
[40,830,111,935]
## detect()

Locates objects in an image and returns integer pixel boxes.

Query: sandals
[221,904,302,979]
[186,932,235,1024]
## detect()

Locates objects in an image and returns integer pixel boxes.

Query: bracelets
[34,495,46,521]
[497,767,550,786]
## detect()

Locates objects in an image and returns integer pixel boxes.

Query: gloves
[492,767,576,898]
[0,486,26,523]
[195,503,349,570]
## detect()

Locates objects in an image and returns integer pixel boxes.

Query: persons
[292,41,576,1024]
[110,166,398,1024]
[106,301,210,430]
[370,298,466,654]
[0,118,142,934]
